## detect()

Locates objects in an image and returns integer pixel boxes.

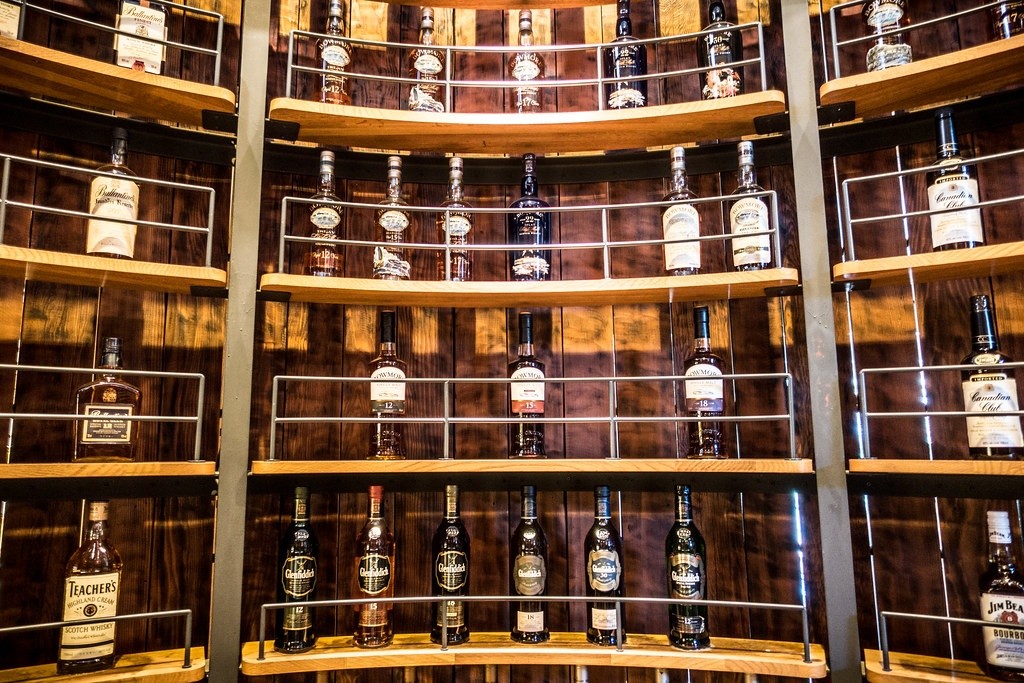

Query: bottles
[860,0,1024,71]
[408,5,447,111]
[660,146,705,276]
[724,141,773,272]
[507,153,552,281]
[508,8,547,113]
[315,0,353,105]
[0,0,170,76]
[604,0,648,110]
[85,128,141,259]
[959,295,1024,460]
[697,0,744,101]
[56,501,123,673]
[70,305,726,459]
[925,109,987,253]
[273,484,710,654]
[436,157,474,281]
[304,150,344,277]
[372,156,415,280]
[979,510,1024,683]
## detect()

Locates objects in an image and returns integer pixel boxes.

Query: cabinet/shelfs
[0,0,1024,683]
